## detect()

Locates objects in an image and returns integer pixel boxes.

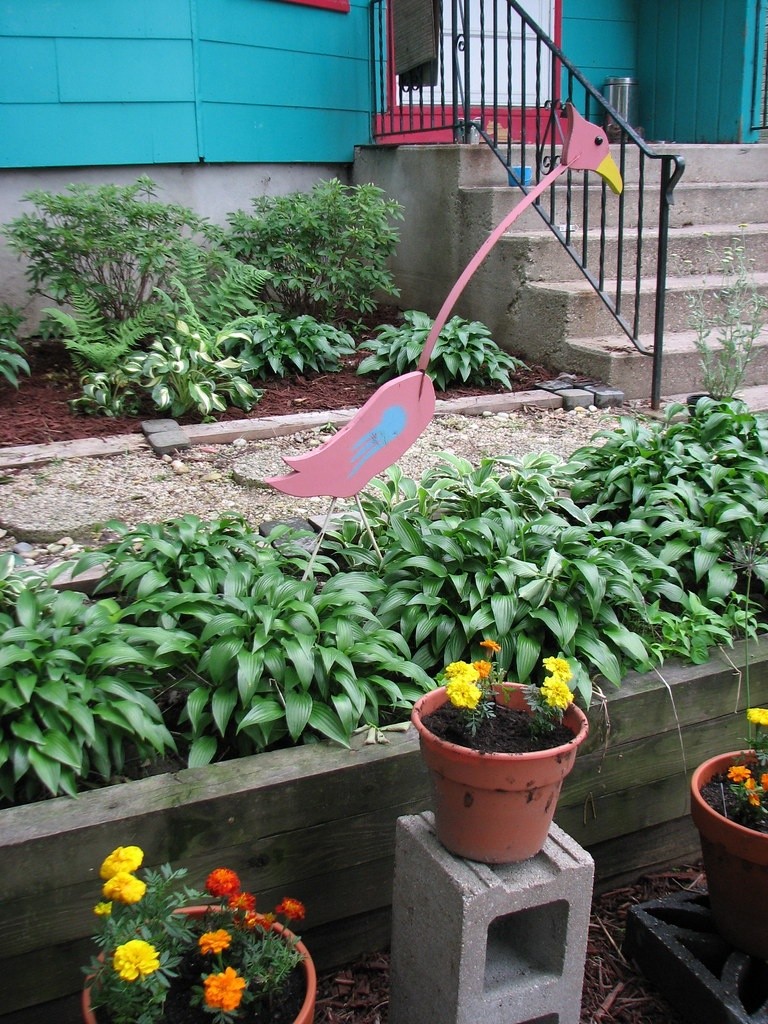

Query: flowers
[443,639,574,737]
[80,846,305,1024]
[726,708,768,805]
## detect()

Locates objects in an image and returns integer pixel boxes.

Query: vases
[691,749,768,956]
[411,682,588,864]
[82,904,316,1023]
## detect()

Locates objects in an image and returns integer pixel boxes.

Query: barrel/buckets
[458,118,481,145]
[508,166,532,186]
